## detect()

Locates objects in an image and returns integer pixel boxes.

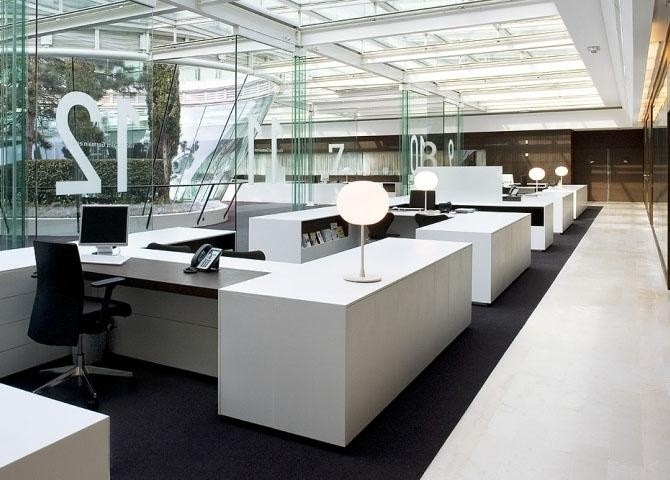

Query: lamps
[529,167,545,197]
[554,166,568,189]
[414,171,438,213]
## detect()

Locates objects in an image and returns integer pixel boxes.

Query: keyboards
[79,255,131,265]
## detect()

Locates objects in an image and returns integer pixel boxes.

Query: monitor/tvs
[410,190,435,211]
[78,204,129,256]
[383,183,396,192]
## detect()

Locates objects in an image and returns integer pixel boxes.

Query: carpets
[1,202,605,480]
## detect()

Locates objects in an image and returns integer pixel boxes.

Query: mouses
[392,207,398,210]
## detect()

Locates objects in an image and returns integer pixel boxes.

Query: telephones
[190,244,223,271]
[509,188,519,195]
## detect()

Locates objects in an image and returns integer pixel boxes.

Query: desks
[0,180,589,480]
